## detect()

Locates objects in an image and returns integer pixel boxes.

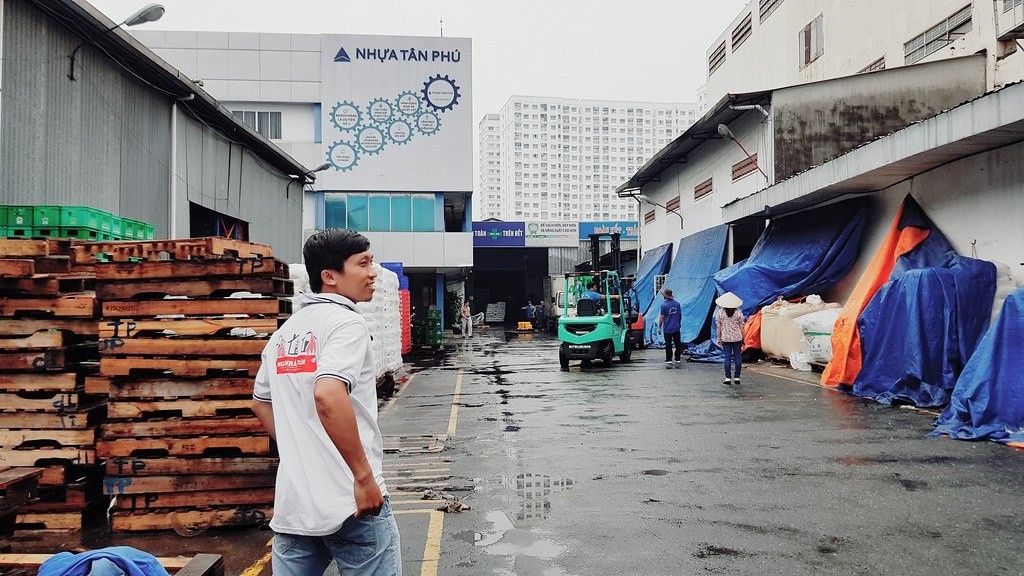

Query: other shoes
[675,361,680,362]
[734,380,740,385]
[469,336,473,338]
[462,336,465,338]
[723,381,731,385]
[664,359,672,363]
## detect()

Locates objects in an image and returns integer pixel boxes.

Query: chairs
[576,299,596,316]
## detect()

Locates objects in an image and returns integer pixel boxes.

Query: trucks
[543,275,577,330]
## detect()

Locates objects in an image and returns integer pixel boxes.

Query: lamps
[717,123,768,182]
[287,162,331,198]
[636,195,684,229]
[70,2,165,80]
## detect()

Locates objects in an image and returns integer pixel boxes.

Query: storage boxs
[380,262,411,354]
[518,322,532,329]
[0,205,155,242]
[411,310,442,345]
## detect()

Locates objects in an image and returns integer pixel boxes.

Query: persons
[249,225,403,576]
[715,291,746,385]
[520,300,546,332]
[659,288,682,368]
[460,300,475,338]
[581,283,621,307]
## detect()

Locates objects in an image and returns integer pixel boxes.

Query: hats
[715,292,743,308]
[528,301,531,303]
[660,288,674,298]
[540,301,544,303]
